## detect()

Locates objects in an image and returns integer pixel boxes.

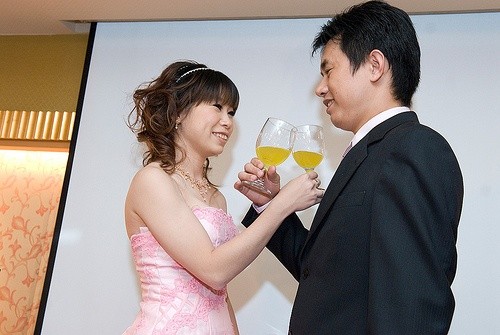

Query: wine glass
[291,125,327,175]
[241,117,298,195]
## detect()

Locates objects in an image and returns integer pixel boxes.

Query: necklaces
[171,161,211,202]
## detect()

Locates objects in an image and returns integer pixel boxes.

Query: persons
[236,1,464,334]
[123,60,324,334]
